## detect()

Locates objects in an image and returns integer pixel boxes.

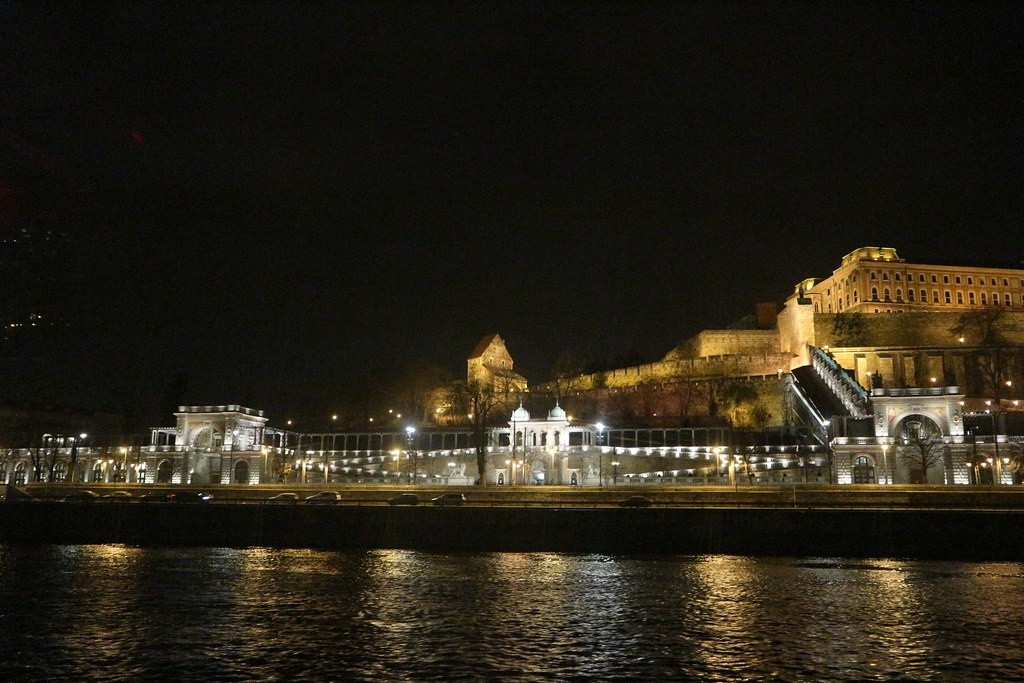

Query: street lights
[592,420,606,487]
[282,420,292,484]
[324,414,338,486]
[823,419,833,484]
[984,409,1000,485]
[404,424,418,484]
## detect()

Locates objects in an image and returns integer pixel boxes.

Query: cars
[63,490,100,503]
[618,496,651,508]
[387,492,418,506]
[100,490,132,503]
[267,493,300,506]
[164,490,214,505]
[430,493,467,507]
[138,492,167,503]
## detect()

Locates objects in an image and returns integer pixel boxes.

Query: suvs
[305,491,342,504]
